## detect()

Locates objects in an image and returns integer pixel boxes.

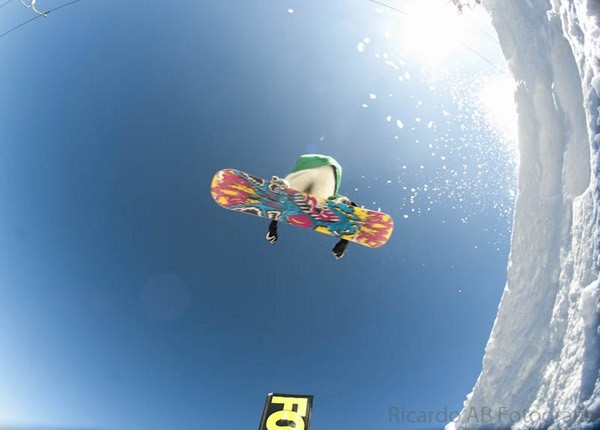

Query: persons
[266,154,354,260]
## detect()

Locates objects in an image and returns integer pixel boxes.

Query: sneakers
[272,176,290,189]
[335,196,352,206]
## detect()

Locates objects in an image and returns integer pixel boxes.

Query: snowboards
[210,169,393,249]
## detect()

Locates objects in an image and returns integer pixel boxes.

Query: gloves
[264,220,278,244]
[329,238,348,259]
